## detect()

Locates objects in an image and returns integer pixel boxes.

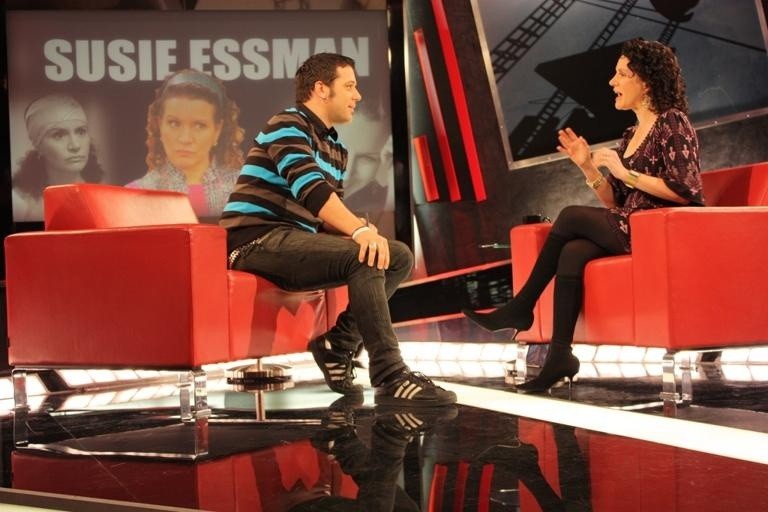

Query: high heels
[462,294,534,341]
[473,436,540,472]
[514,338,580,392]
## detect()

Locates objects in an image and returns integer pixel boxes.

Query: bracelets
[584,173,604,188]
[350,225,371,239]
[624,168,640,190]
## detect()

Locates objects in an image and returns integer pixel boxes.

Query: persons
[120,68,249,217]
[286,383,459,512]
[215,52,457,409]
[471,421,590,510]
[11,90,106,224]
[460,36,706,393]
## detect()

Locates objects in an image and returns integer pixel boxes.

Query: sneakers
[312,394,365,455]
[372,408,459,445]
[308,333,365,394]
[374,366,458,407]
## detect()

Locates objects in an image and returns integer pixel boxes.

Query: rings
[369,242,377,248]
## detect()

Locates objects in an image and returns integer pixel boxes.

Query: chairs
[502,162,768,417]
[0,183,349,464]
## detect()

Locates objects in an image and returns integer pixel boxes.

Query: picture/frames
[467,0,768,172]
